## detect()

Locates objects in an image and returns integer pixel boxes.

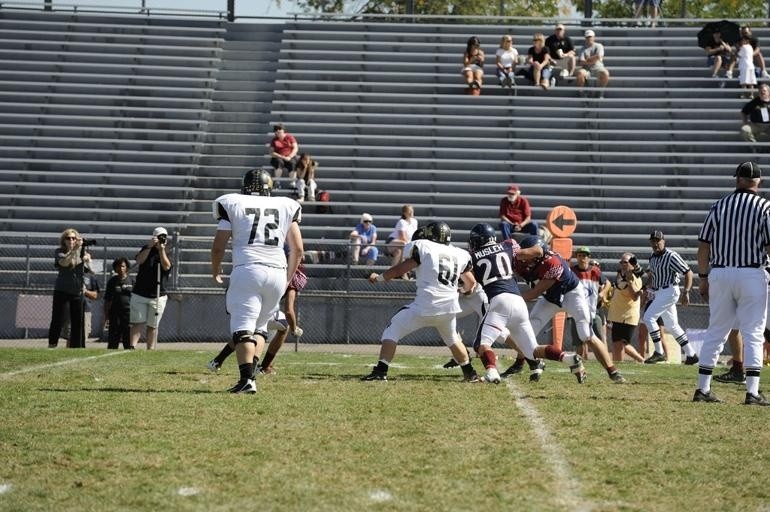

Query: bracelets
[145,245,151,251]
[289,155,293,159]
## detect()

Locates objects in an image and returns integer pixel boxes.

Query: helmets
[520,237,549,255]
[243,168,273,196]
[470,223,496,247]
[424,221,451,245]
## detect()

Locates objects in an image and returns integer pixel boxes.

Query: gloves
[291,326,304,338]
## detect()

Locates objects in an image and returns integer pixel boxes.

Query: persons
[494,35,519,88]
[48,228,86,348]
[545,23,576,77]
[705,25,770,99]
[270,125,298,189]
[294,152,318,201]
[738,83,769,153]
[349,183,702,383]
[100,257,137,349]
[573,30,609,98]
[129,227,173,350]
[83,251,99,348]
[525,33,551,90]
[691,163,770,406]
[460,37,485,96]
[206,166,307,394]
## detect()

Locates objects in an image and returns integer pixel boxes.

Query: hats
[575,246,591,253]
[734,160,761,178]
[555,24,564,30]
[153,227,168,236]
[505,185,518,193]
[649,230,664,240]
[584,30,595,36]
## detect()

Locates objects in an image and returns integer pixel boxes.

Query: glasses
[619,261,629,264]
[66,237,75,241]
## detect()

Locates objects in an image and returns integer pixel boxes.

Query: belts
[711,264,761,267]
[651,281,677,291]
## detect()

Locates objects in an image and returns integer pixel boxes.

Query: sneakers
[541,83,548,92]
[685,354,699,365]
[501,365,524,377]
[608,370,626,383]
[713,368,746,385]
[461,370,479,383]
[530,360,545,382]
[361,366,388,382]
[470,80,481,96]
[470,371,501,384]
[502,78,511,88]
[208,360,219,371]
[227,378,257,394]
[567,354,587,383]
[693,389,725,403]
[263,367,275,374]
[443,357,471,369]
[745,392,770,406]
[644,351,666,363]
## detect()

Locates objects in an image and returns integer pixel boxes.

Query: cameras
[158,234,167,244]
[80,238,96,259]
[629,257,644,277]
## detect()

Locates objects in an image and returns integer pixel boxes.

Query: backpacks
[316,188,333,212]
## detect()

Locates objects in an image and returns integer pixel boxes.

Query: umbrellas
[695,20,741,49]
